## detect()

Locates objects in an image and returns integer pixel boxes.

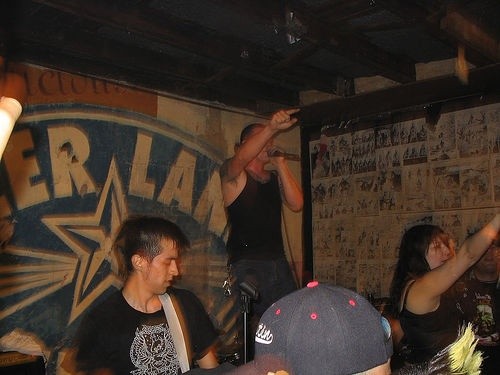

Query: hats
[225,281,393,375]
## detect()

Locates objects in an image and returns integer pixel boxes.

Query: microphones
[268,151,300,161]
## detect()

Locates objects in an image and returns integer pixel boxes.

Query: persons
[221,106,305,325]
[391,215,500,375]
[72,217,220,375]
[223,281,392,375]
